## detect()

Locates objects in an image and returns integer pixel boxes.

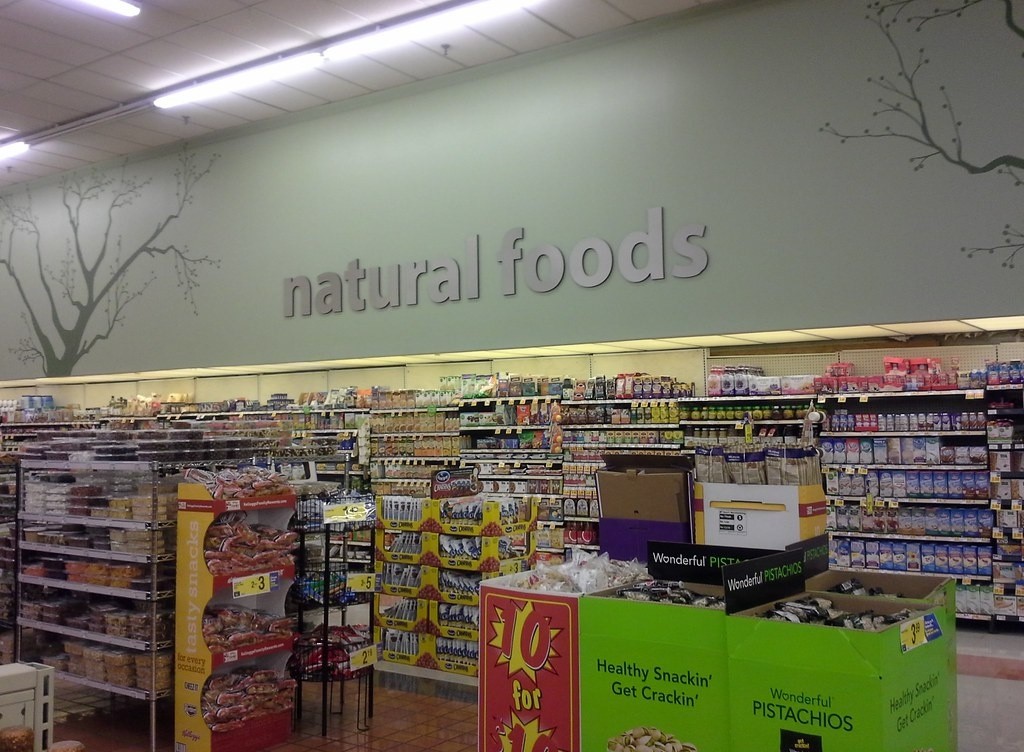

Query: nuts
[606,726,697,752]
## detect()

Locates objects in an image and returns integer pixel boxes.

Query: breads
[184,466,297,733]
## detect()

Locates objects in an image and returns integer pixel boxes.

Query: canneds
[693,427,728,438]
[21,395,53,410]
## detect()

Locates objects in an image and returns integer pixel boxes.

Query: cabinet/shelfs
[371,388,460,497]
[678,394,818,457]
[815,390,996,635]
[458,394,565,570]
[0,384,377,752]
[987,383,1024,627]
[558,397,688,561]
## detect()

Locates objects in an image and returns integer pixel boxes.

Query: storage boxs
[372,465,597,679]
[821,466,1024,621]
[593,454,695,565]
[693,480,828,550]
[785,533,957,751]
[721,551,953,751]
[578,540,789,752]
[476,559,644,752]
